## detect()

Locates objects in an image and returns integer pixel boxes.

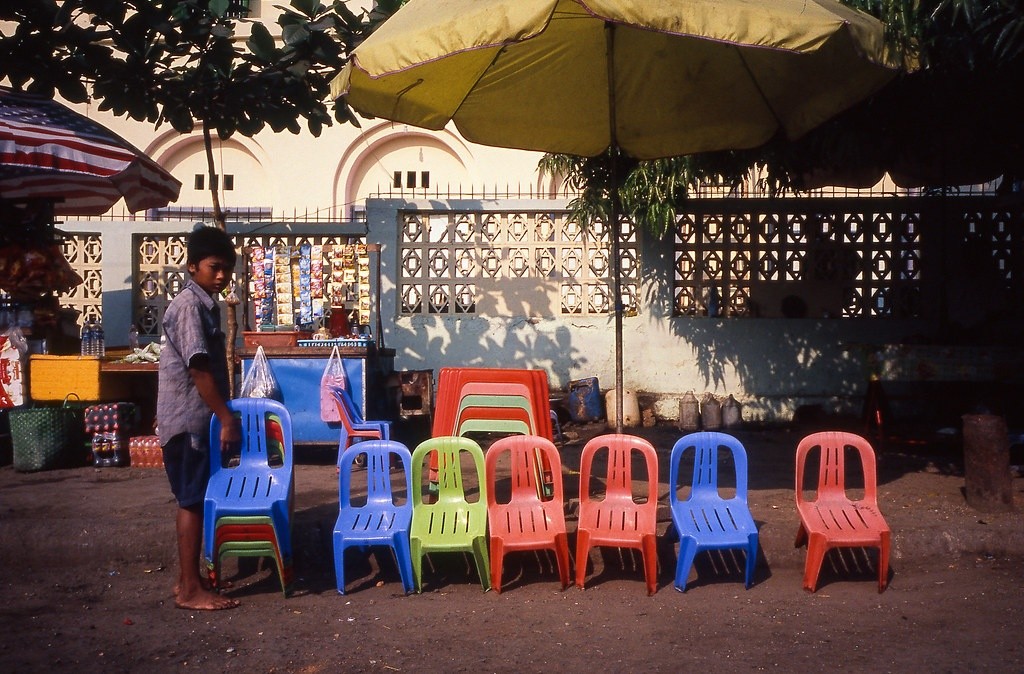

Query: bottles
[84,402,162,468]
[91,321,105,356]
[722,394,741,428]
[81,321,91,356]
[700,392,720,429]
[679,391,699,429]
[128,325,139,352]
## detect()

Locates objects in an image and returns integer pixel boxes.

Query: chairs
[202,397,294,598]
[325,384,396,471]
[668,432,760,593]
[575,434,659,597]
[795,432,892,592]
[332,435,571,596]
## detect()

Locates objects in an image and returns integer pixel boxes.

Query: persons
[157,227,242,610]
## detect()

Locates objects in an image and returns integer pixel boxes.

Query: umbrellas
[328,0,888,435]
[0,84,183,215]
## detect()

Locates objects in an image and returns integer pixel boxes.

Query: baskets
[9,394,92,472]
[241,331,312,347]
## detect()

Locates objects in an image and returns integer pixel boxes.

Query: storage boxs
[29,354,128,402]
[240,325,312,348]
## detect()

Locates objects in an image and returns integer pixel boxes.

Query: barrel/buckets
[563,377,601,421]
[605,389,640,429]
[549,393,565,409]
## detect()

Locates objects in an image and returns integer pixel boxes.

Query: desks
[428,366,553,497]
[101,355,240,435]
